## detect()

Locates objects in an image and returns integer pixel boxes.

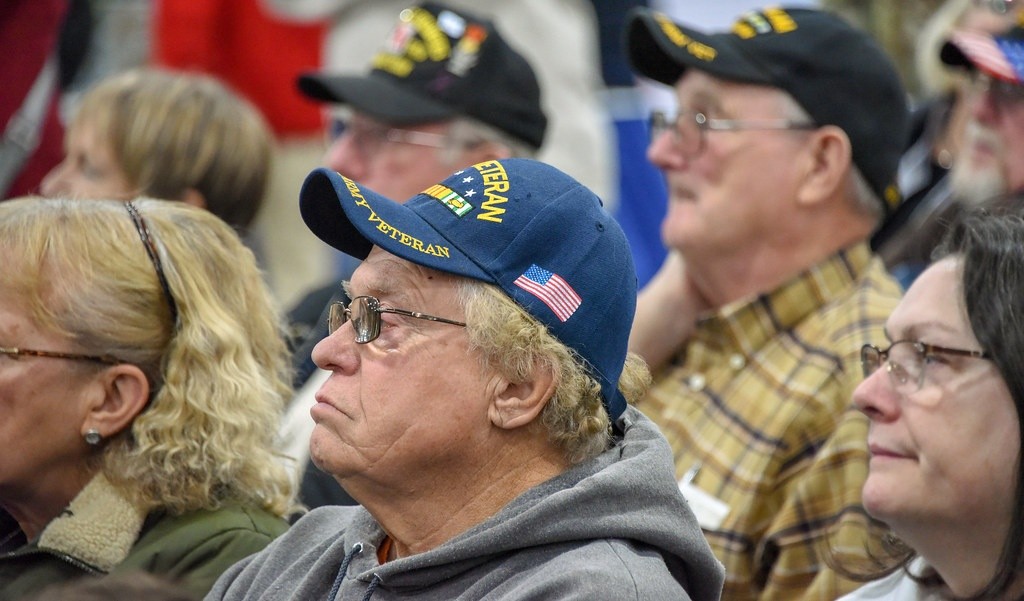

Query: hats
[941,34,1023,83]
[299,154,637,425]
[627,4,909,217]
[294,1,546,147]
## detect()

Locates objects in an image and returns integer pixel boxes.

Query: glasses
[861,339,997,399]
[319,107,486,163]
[643,107,820,159]
[327,297,468,346]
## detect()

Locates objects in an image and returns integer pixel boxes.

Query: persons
[0,0,1024,526]
[0,196,299,601]
[204,158,727,601]
[618,6,913,601]
[832,205,1022,600]
